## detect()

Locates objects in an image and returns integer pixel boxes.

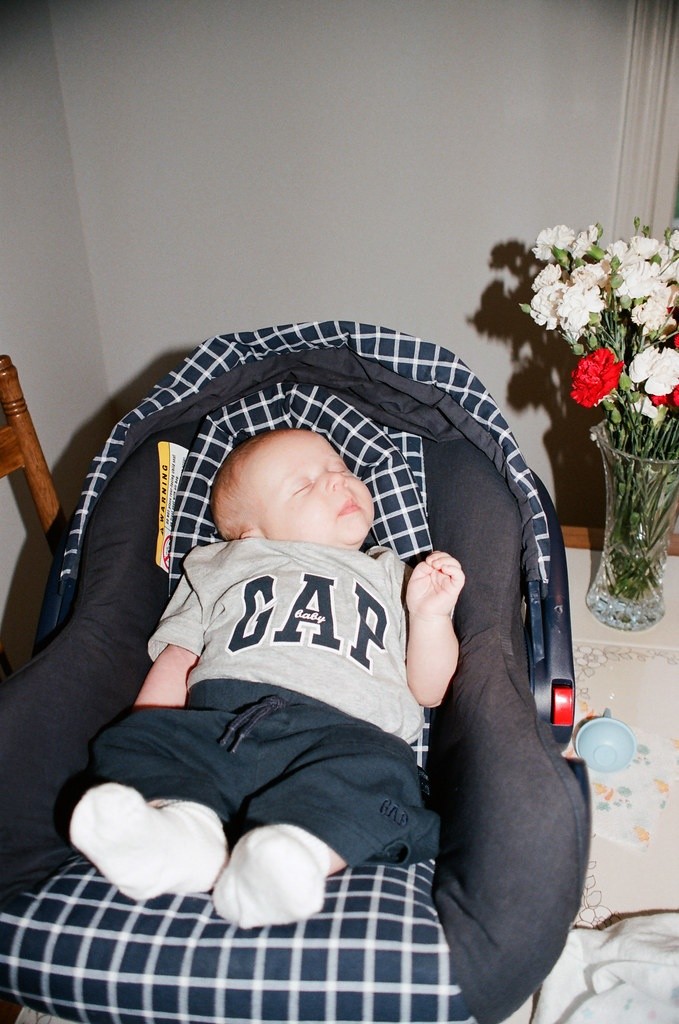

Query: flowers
[516,218,679,602]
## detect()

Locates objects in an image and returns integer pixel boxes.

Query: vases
[584,421,679,630]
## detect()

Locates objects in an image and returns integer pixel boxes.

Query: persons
[70,427,466,928]
